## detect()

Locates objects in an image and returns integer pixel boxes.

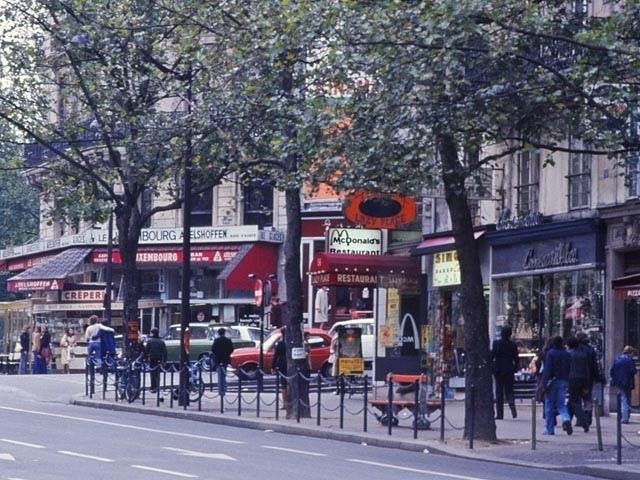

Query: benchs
[369,398,442,429]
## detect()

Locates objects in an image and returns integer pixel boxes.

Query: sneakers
[494,407,517,419]
[542,421,628,435]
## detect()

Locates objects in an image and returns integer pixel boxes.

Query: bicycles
[114,352,145,403]
[172,352,210,404]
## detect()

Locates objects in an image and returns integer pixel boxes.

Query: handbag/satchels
[12,341,24,353]
[582,384,592,402]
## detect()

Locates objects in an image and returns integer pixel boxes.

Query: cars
[49,317,83,332]
[142,317,376,380]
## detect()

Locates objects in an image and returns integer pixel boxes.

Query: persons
[147,327,167,392]
[329,326,346,395]
[86,314,115,364]
[490,325,520,420]
[271,328,288,411]
[610,345,635,423]
[90,318,115,358]
[303,331,312,371]
[212,328,233,391]
[138,330,147,385]
[313,286,331,329]
[534,329,596,435]
[20,323,76,375]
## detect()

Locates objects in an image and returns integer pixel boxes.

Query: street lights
[248,271,277,391]
[94,148,124,327]
